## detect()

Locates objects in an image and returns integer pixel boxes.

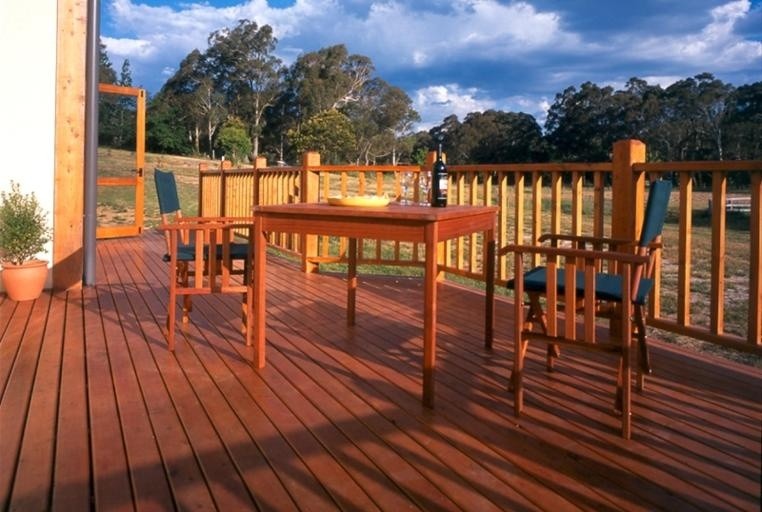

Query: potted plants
[0,177,61,305]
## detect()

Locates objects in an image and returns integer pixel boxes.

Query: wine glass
[398,171,432,208]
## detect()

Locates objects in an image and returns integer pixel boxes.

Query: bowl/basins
[325,195,392,208]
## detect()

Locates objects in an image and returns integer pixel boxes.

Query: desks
[249,200,500,410]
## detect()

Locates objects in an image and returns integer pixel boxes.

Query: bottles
[433,143,449,208]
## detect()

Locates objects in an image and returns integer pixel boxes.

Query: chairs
[494,179,672,444]
[153,168,260,354]
